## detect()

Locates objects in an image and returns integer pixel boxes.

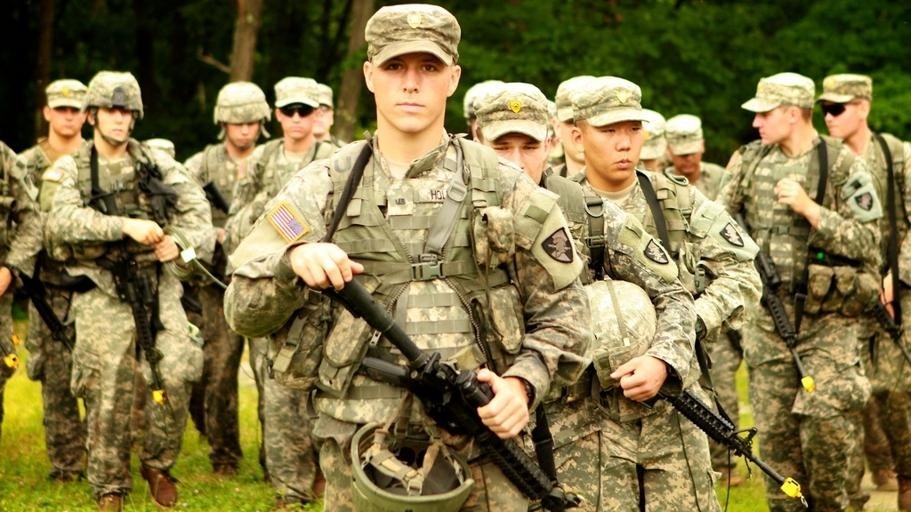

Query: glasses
[278,105,315,119]
[820,100,862,117]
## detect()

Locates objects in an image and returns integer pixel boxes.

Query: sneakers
[897,470,911,511]
[97,490,128,512]
[212,462,243,478]
[273,493,306,511]
[716,464,743,488]
[50,469,84,484]
[310,468,327,500]
[140,458,180,508]
[870,465,901,492]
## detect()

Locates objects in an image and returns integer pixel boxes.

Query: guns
[733,208,807,381]
[658,380,785,487]
[13,260,75,351]
[319,280,582,511]
[874,298,910,368]
[88,187,164,371]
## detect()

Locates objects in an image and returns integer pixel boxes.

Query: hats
[363,3,465,69]
[817,73,874,105]
[738,71,817,114]
[556,73,645,127]
[664,113,705,158]
[273,74,334,109]
[42,76,89,112]
[461,79,556,144]
[639,108,668,162]
[142,137,178,159]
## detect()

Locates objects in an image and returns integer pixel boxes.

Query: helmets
[213,79,273,126]
[348,417,478,511]
[581,278,658,396]
[81,68,146,121]
